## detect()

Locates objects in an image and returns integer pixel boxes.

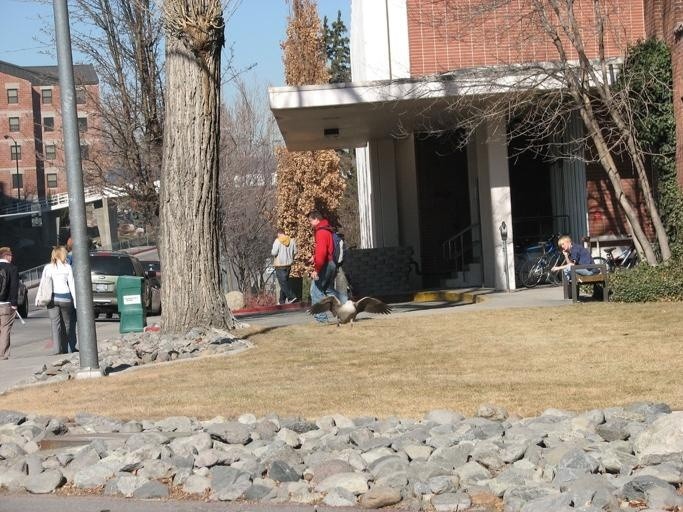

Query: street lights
[2,134,21,201]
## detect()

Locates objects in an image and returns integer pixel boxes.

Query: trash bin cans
[117,274,147,334]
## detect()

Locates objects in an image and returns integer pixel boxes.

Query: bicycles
[518,230,564,289]
[588,231,637,275]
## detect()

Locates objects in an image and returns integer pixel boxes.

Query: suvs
[85,245,161,319]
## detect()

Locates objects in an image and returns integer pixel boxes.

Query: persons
[551,235,600,284]
[0,236,79,360]
[271,211,348,322]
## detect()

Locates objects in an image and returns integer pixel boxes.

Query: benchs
[560,256,609,304]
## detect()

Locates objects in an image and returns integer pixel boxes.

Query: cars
[14,271,30,320]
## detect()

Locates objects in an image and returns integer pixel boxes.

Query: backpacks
[319,226,344,265]
[0,267,9,301]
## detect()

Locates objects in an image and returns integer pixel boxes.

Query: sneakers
[289,297,297,304]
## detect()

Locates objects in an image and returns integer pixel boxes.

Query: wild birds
[305,294,393,328]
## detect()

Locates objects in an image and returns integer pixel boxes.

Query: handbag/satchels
[35,278,52,308]
